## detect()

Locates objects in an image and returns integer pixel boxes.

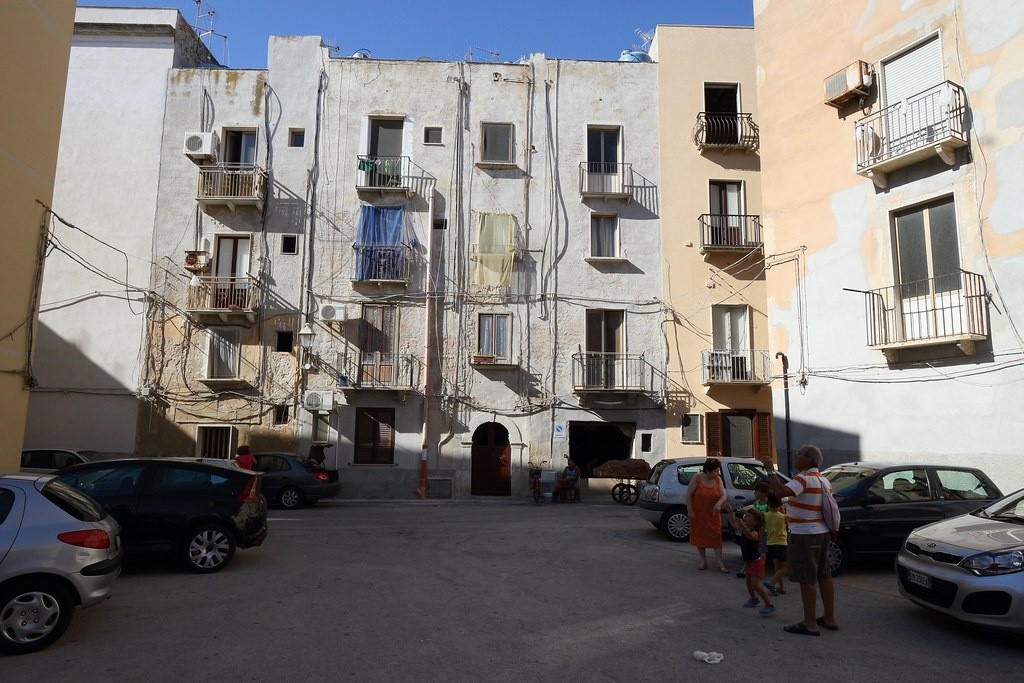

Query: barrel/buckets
[544,493,553,504]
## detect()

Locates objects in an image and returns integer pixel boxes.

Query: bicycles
[528,461,547,502]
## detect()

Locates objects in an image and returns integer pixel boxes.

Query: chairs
[121,476,132,490]
[65,457,74,467]
[555,479,572,502]
[872,478,912,502]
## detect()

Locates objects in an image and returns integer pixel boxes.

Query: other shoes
[716,563,729,572]
[698,562,707,569]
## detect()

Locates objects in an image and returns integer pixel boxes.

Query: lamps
[298,322,317,357]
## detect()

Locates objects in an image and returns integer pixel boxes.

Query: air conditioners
[304,390,334,411]
[319,303,348,323]
[703,351,732,381]
[182,131,219,159]
[184,251,209,271]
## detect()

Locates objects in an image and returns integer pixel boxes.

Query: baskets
[530,469,542,478]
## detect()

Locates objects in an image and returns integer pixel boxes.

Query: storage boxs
[822,59,868,105]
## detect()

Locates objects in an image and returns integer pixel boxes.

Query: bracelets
[767,470,776,475]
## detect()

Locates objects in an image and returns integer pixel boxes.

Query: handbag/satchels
[821,480,840,532]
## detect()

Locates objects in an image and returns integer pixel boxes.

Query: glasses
[797,450,801,455]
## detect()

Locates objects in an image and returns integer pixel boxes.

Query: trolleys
[593,458,652,505]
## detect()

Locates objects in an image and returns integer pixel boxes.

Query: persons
[563,459,580,500]
[724,482,790,614]
[687,458,729,572]
[761,445,840,636]
[234,445,257,471]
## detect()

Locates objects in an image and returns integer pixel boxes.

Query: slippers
[759,605,775,614]
[764,582,777,595]
[816,617,838,631]
[776,588,785,594]
[744,598,760,608]
[783,622,821,636]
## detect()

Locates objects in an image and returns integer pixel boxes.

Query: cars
[896,489,1023,634]
[49,456,268,573]
[21,449,112,484]
[728,460,1007,576]
[252,441,342,510]
[0,473,124,655]
[637,456,793,541]
